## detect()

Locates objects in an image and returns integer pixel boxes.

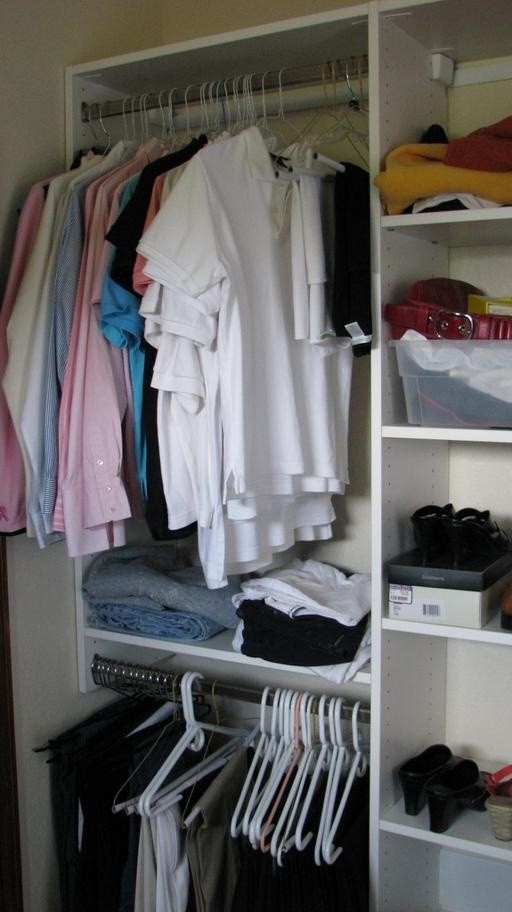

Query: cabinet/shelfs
[64,0,512,912]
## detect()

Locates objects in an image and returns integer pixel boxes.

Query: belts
[385,278,511,341]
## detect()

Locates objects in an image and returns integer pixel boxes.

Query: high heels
[398,744,479,833]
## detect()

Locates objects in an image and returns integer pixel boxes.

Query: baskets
[485,795,512,841]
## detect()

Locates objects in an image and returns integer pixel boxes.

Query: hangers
[34,653,368,866]
[79,55,368,175]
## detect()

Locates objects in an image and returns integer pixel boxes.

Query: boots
[411,503,512,568]
